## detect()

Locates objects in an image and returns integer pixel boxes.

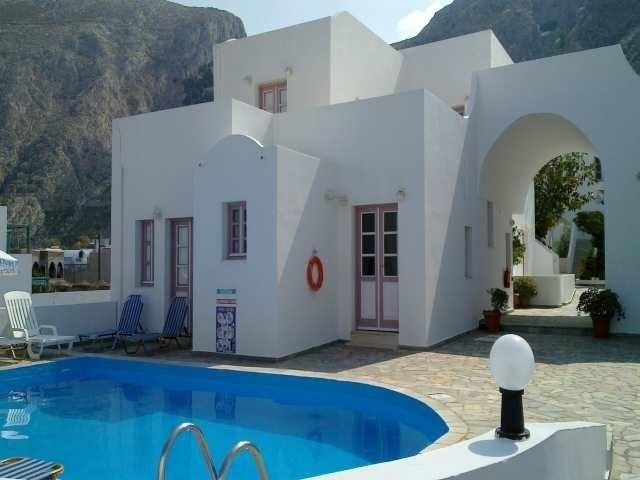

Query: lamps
[490,334,535,439]
[396,190,406,203]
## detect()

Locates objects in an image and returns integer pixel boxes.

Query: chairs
[0,290,189,361]
[4,408,30,427]
[0,455,65,480]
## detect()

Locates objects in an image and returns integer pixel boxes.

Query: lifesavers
[306,256,323,291]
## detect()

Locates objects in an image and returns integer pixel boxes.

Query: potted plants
[577,287,626,338]
[483,287,509,331]
[514,277,537,308]
